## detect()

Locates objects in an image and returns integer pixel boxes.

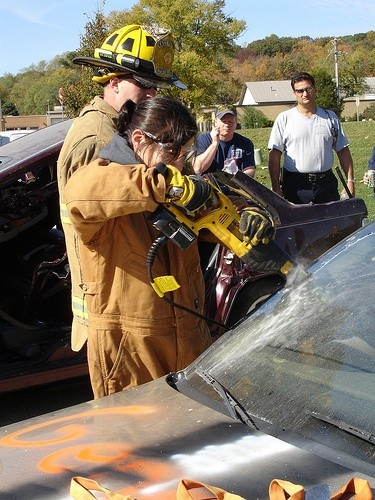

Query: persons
[192,104,257,272]
[57,24,188,351]
[364,147,375,198]
[64,98,274,400]
[267,72,356,205]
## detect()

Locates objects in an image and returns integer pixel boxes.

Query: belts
[284,168,332,181]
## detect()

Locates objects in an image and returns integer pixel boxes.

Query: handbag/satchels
[279,166,285,185]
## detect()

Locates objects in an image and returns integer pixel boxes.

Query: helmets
[72,24,188,90]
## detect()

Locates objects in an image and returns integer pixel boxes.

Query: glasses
[295,85,315,93]
[144,129,198,162]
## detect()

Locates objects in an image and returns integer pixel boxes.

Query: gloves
[165,164,220,217]
[239,207,275,246]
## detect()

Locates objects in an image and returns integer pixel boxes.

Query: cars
[0,117,369,397]
[0,217,375,500]
[0,129,36,147]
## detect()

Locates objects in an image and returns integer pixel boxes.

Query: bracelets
[347,179,355,182]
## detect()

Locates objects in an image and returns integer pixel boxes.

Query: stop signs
[58,88,65,104]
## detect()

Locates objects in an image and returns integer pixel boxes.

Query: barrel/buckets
[254,149,264,165]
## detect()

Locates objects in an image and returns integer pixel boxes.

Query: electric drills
[150,177,309,277]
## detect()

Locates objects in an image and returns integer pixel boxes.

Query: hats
[216,104,238,119]
